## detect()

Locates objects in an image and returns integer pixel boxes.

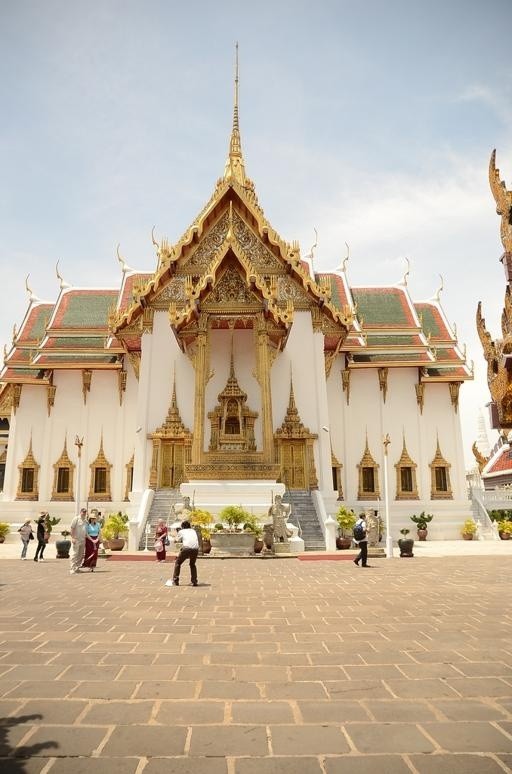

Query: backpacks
[354,521,366,541]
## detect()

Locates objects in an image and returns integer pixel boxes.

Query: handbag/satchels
[165,539,170,545]
[44,532,48,543]
[29,533,34,540]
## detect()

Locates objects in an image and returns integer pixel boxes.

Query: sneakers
[353,560,358,566]
[362,565,370,567]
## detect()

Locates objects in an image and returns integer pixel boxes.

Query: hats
[25,518,30,523]
[40,511,47,516]
[90,514,96,518]
[154,540,163,552]
[81,508,86,512]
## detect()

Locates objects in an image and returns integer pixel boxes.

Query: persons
[154,519,168,563]
[79,513,101,572]
[172,520,199,586]
[353,513,371,568]
[68,509,87,573]
[20,519,35,561]
[34,513,47,562]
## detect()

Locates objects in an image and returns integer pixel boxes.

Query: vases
[101,541,109,549]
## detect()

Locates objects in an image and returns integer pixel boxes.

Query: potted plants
[0,521,10,543]
[409,511,433,541]
[334,504,357,550]
[187,509,210,554]
[209,505,256,557]
[461,517,477,540]
[103,512,132,551]
[253,527,264,553]
[498,521,512,540]
